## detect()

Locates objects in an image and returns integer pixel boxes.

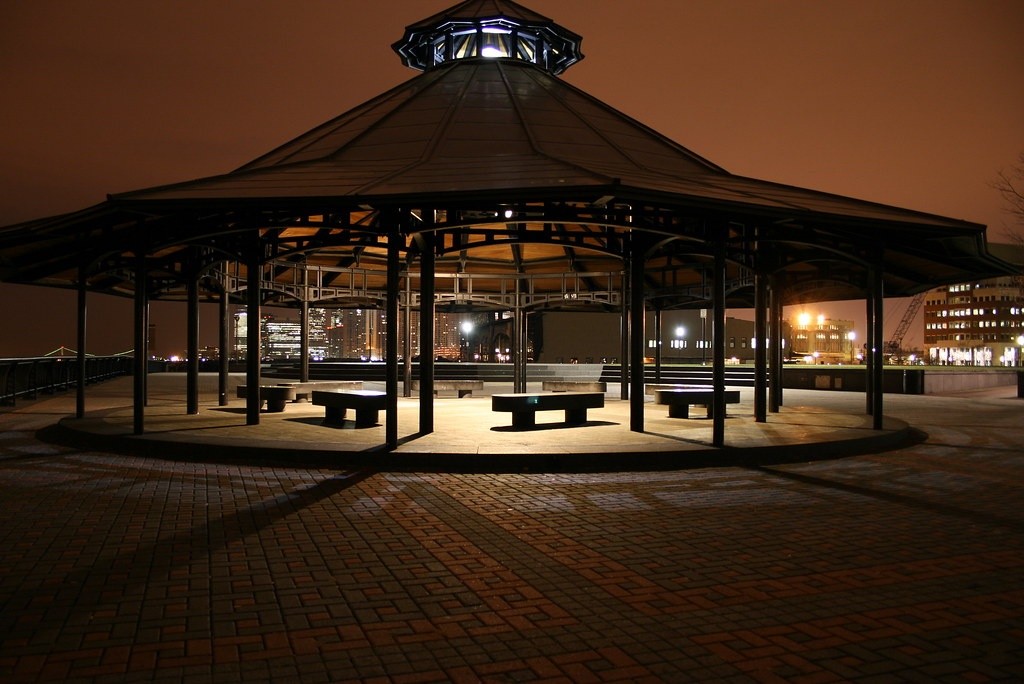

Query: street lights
[462,322,472,362]
[233,315,241,365]
[813,352,818,365]
[1017,336,1024,366]
[701,309,707,364]
[848,332,855,365]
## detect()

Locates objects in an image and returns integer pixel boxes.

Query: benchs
[654,389,740,418]
[411,380,483,399]
[492,392,605,426]
[312,389,387,425]
[645,384,726,408]
[543,381,607,393]
[277,380,363,403]
[236,384,296,412]
